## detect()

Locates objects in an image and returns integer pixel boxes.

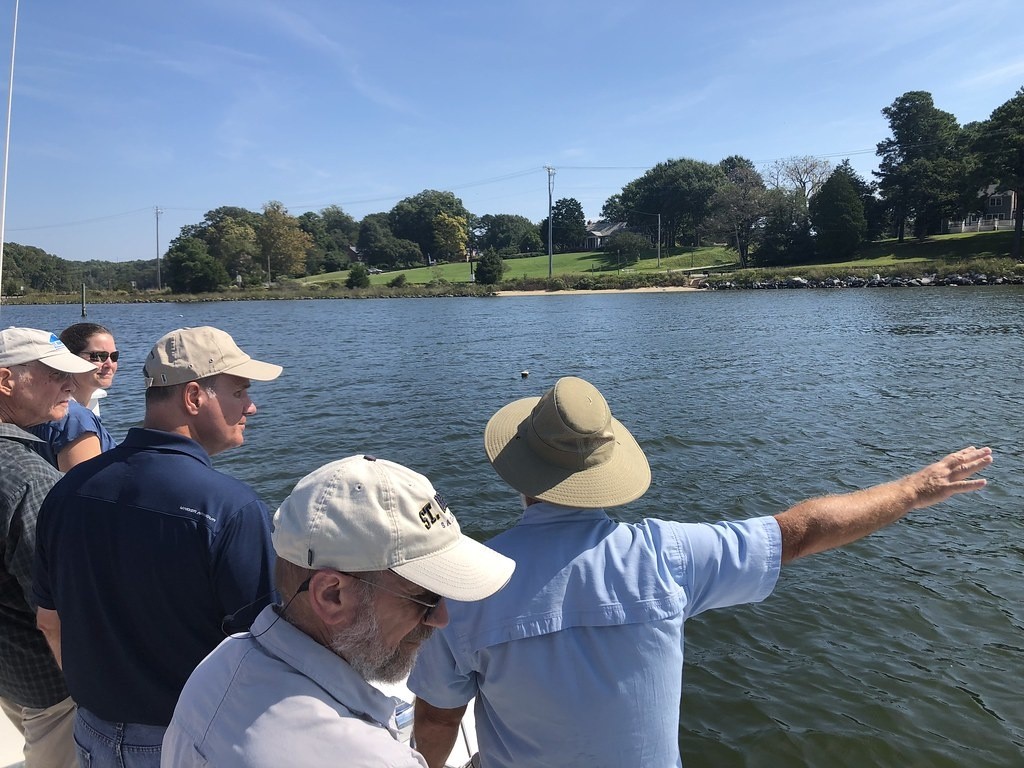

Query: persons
[0,324,994,768]
[161,455,516,768]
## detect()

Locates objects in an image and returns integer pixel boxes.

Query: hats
[143,324,283,387]
[0,326,99,377]
[484,376,651,509]
[271,456,516,603]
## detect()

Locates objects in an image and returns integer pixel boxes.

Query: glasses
[19,364,72,382]
[338,571,442,623]
[80,350,119,362]
